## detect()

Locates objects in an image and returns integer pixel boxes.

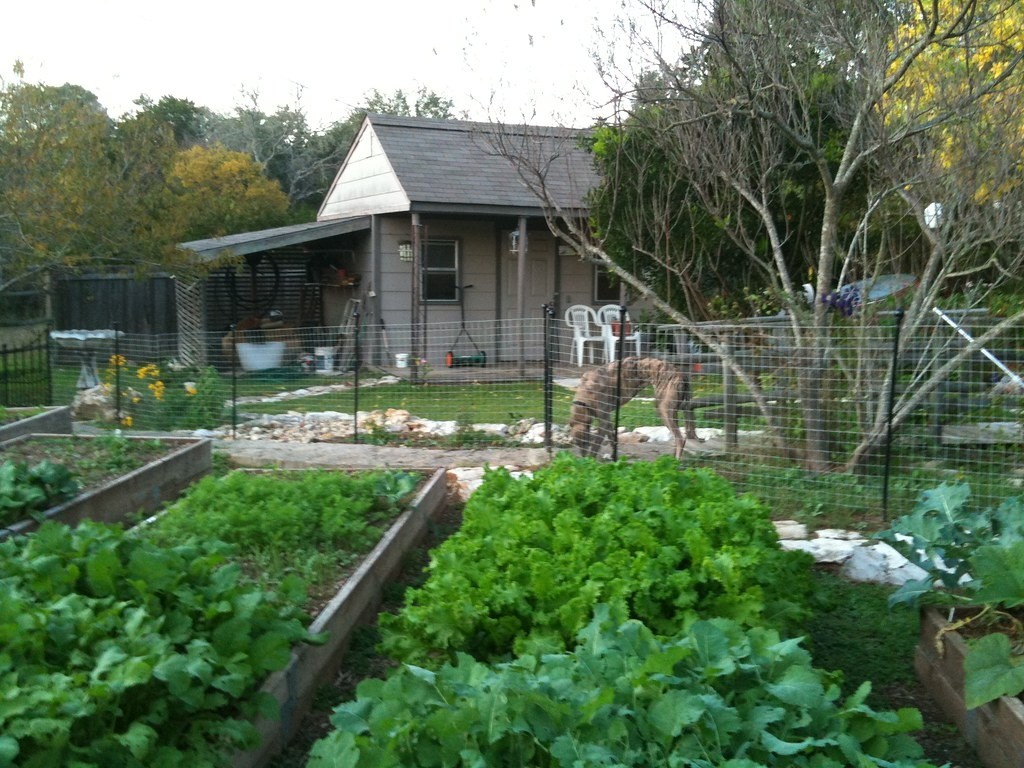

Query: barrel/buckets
[395,354,409,368]
[314,347,334,374]
[235,340,286,373]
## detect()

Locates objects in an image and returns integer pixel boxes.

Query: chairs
[596,304,641,364]
[565,304,609,368]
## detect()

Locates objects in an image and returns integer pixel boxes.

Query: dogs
[570,358,691,462]
